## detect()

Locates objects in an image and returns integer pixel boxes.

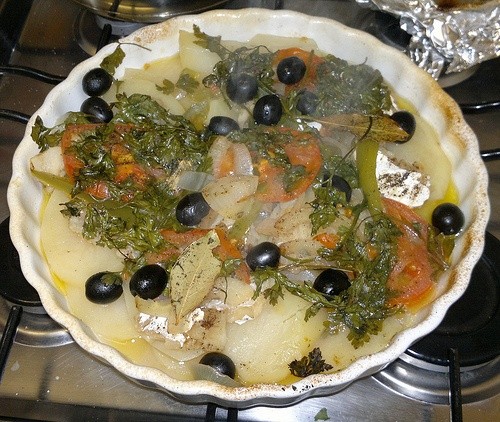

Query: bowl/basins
[8,7,491,409]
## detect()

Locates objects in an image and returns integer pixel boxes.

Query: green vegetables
[31,26,465,377]
